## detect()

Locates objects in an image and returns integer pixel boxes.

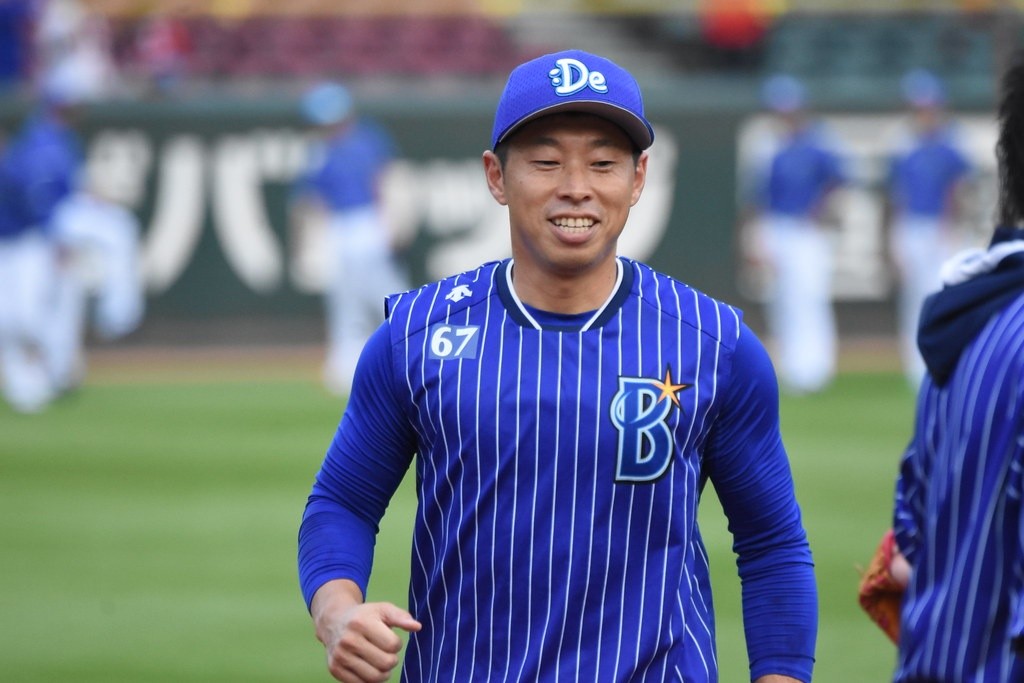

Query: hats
[490,51,654,154]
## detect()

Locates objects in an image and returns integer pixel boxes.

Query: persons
[761,108,847,389]
[858,36,1023,683]
[296,50,820,683]
[288,95,409,391]
[1,94,147,414]
